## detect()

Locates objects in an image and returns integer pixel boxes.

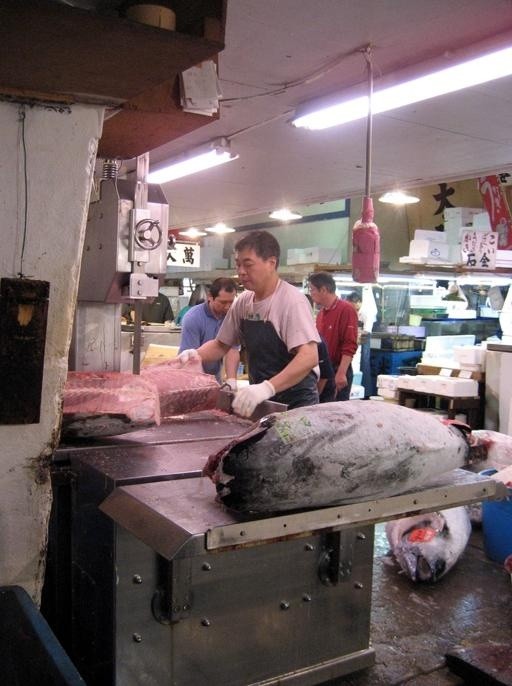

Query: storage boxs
[376,371,479,400]
[287,247,342,267]
[409,240,449,261]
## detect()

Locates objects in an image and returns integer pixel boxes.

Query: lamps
[143,134,240,184]
[377,188,421,206]
[268,208,303,220]
[285,27,511,135]
[178,221,237,237]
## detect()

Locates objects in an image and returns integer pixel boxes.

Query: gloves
[225,377,236,391]
[232,380,275,418]
[175,349,198,362]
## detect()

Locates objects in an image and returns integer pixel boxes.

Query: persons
[305,271,358,404]
[176,276,241,393]
[174,284,209,327]
[172,229,324,423]
[121,292,175,328]
[303,295,333,402]
[347,293,370,349]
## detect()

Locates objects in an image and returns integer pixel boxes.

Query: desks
[369,345,425,397]
